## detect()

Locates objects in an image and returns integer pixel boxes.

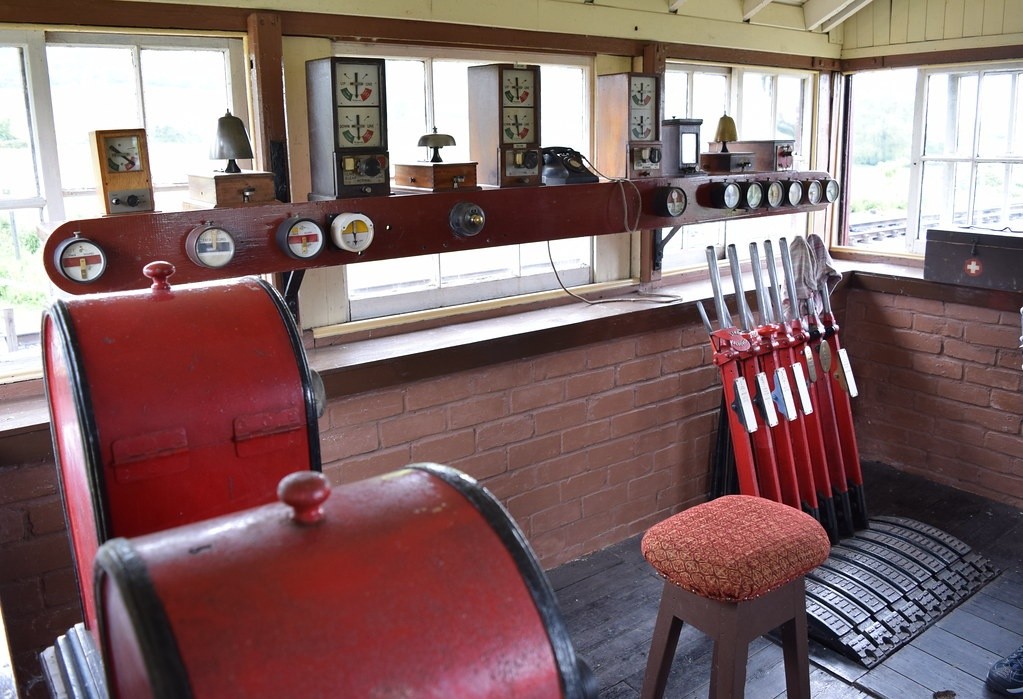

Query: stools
[641,494,829,699]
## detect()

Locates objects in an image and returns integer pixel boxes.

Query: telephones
[542,146,600,186]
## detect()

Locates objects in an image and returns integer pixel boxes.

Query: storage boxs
[700,153,756,173]
[393,160,480,190]
[187,171,276,206]
[922,226,1023,292]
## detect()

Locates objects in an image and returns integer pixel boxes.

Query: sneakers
[986,644,1023,699]
